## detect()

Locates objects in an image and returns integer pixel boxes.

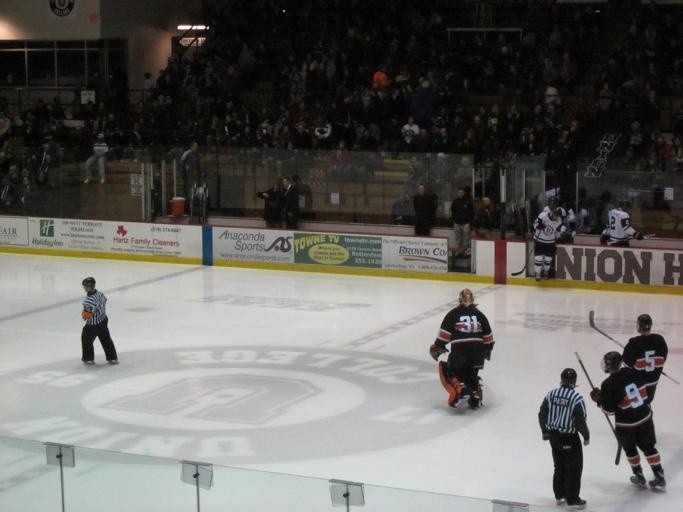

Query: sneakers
[555,494,586,505]
[631,475,665,486]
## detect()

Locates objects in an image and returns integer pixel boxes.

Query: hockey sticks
[576,351,622,465]
[511,229,541,275]
[589,310,680,385]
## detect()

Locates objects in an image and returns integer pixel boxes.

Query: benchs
[76,147,414,220]
[603,167,683,231]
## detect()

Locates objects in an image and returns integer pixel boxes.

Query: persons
[0,1,682,248]
[431,288,495,410]
[531,209,566,282]
[589,352,667,489]
[537,367,592,505]
[81,277,119,367]
[622,315,667,406]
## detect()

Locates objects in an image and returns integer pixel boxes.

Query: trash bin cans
[169,197,186,218]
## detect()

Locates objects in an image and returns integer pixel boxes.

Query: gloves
[590,388,602,401]
[633,231,643,240]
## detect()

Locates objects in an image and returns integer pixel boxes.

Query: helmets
[561,368,576,384]
[459,289,472,304]
[604,352,621,370]
[638,314,652,331]
[83,278,95,287]
